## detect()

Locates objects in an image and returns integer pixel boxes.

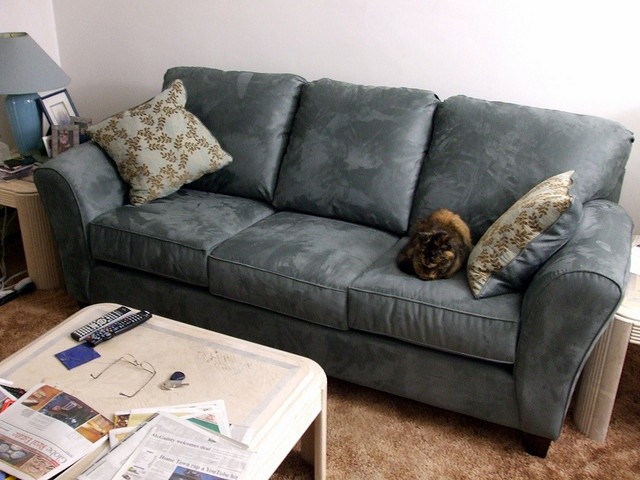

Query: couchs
[33,66,633,459]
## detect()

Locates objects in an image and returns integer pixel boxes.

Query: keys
[160,372,190,390]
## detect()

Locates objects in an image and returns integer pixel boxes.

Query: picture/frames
[52,125,79,157]
[37,89,80,126]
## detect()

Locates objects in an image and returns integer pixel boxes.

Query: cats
[396,209,471,281]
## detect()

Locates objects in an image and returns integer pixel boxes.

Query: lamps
[0,31,71,158]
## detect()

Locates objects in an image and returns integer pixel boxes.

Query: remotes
[86,310,152,347]
[70,306,132,342]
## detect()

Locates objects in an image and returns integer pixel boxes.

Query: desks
[573,232,640,444]
[0,165,62,292]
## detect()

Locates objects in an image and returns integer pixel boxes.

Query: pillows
[84,79,232,204]
[467,170,583,300]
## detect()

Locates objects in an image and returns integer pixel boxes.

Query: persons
[59,129,73,150]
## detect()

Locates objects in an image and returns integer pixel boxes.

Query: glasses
[90,353,156,398]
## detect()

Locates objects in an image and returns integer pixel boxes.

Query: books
[1,155,36,181]
